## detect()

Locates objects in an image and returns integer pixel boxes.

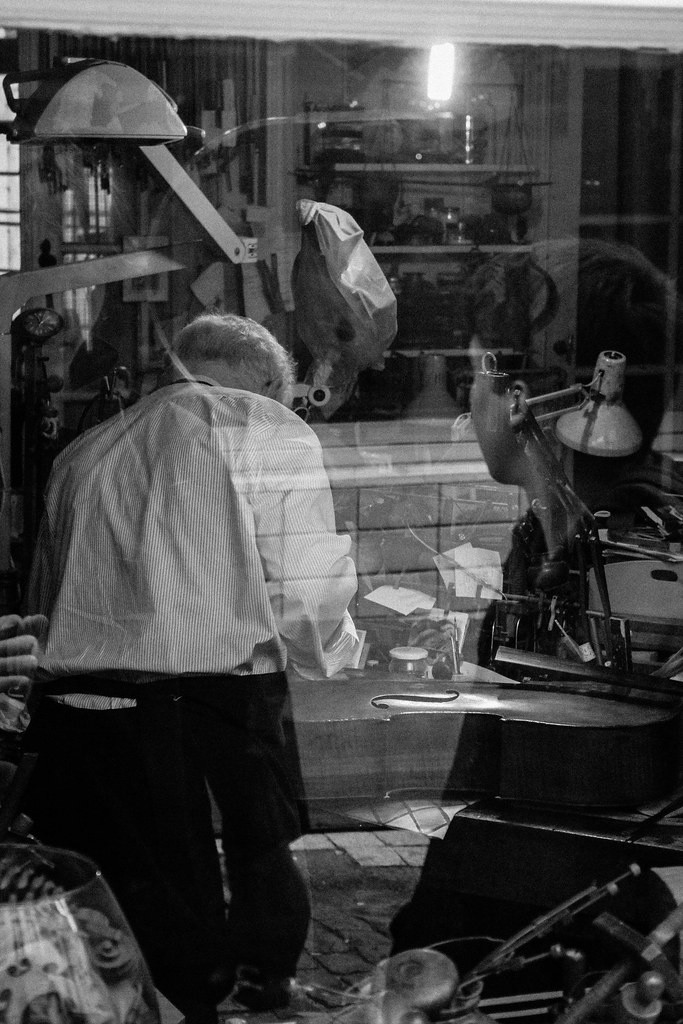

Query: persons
[376,238,683,1024]
[25,313,377,1020]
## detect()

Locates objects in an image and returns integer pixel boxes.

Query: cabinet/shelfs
[332,107,545,415]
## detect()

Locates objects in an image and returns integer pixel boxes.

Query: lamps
[512,350,646,698]
